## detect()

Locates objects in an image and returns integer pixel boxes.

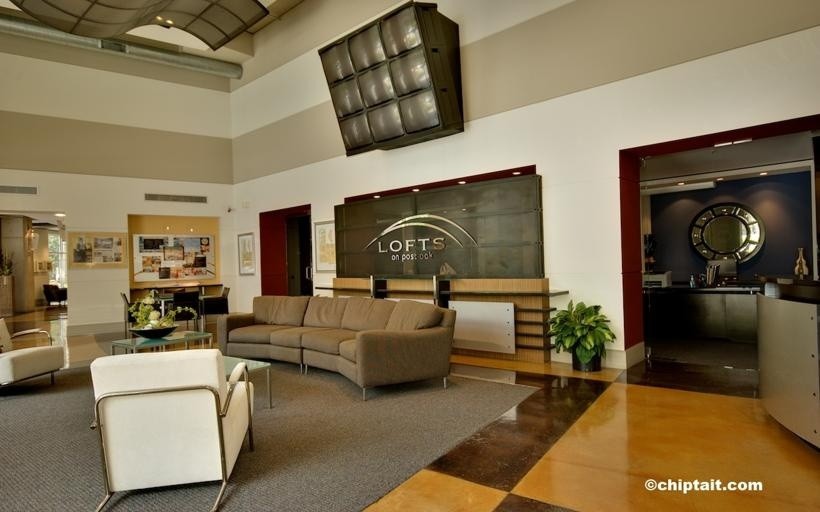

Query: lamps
[640,181,717,197]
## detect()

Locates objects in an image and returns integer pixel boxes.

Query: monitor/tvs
[318,0,464,157]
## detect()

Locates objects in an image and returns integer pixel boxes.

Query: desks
[0,275,15,334]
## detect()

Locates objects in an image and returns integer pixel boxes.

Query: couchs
[216,305,457,401]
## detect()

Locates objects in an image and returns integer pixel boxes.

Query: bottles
[689,274,696,288]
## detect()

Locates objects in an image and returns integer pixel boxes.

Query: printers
[642,271,672,288]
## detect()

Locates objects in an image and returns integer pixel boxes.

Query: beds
[222,355,273,409]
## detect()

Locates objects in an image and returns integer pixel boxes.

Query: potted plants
[544,299,617,372]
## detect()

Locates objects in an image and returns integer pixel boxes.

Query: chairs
[119,285,230,354]
[90,346,255,512]
[42,284,67,308]
[0,318,65,386]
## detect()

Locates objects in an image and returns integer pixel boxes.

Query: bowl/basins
[129,326,180,341]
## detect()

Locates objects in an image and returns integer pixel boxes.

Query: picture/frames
[237,232,256,276]
[66,229,128,270]
[314,220,336,273]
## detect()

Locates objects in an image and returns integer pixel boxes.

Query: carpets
[0,351,541,511]
[651,337,758,372]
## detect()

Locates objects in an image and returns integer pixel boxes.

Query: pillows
[253,294,442,332]
